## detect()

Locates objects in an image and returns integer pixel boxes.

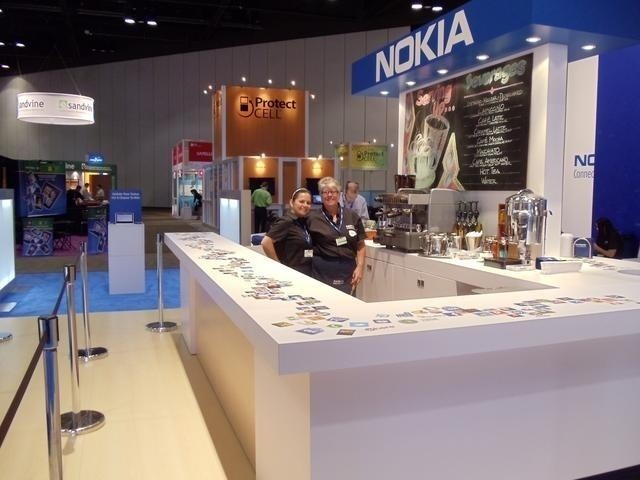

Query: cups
[427,231,460,257]
[423,113,450,170]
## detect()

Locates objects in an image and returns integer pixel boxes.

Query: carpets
[0,265,180,318]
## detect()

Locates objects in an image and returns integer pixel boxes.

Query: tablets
[40,182,63,209]
[32,193,43,210]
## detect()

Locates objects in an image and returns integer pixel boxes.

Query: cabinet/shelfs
[355,256,477,304]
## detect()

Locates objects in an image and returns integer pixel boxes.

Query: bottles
[455,218,481,249]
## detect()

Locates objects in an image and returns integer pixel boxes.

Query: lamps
[17,49,96,127]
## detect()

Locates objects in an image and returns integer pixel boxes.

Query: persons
[25,172,57,212]
[593,216,624,258]
[338,180,371,228]
[93,184,104,202]
[260,186,313,277]
[251,182,273,233]
[67,185,84,207]
[190,189,203,220]
[82,183,93,199]
[305,176,367,297]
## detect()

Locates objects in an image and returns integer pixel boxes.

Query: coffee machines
[368,189,461,252]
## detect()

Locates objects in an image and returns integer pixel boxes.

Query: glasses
[322,190,338,194]
